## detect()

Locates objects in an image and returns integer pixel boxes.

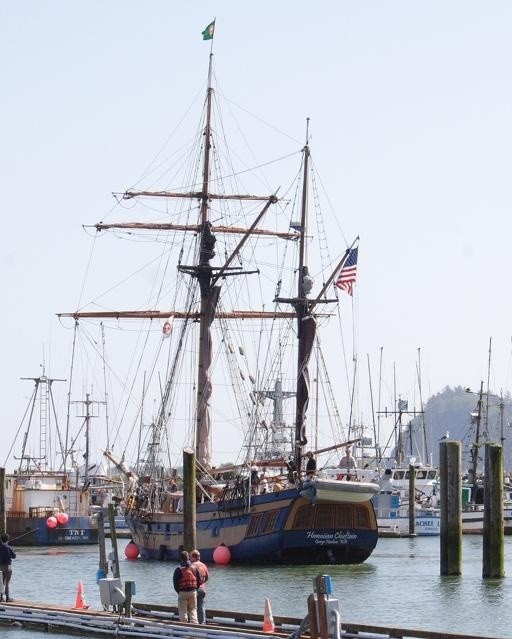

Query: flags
[335,246,358,298]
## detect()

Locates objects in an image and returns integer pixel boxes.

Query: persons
[244,451,316,498]
[0,534,16,601]
[190,549,209,624]
[172,550,201,624]
[169,480,178,510]
[338,448,357,482]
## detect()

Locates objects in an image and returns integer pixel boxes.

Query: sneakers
[1,595,14,602]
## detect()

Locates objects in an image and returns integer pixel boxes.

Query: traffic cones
[73,581,88,608]
[258,597,278,632]
